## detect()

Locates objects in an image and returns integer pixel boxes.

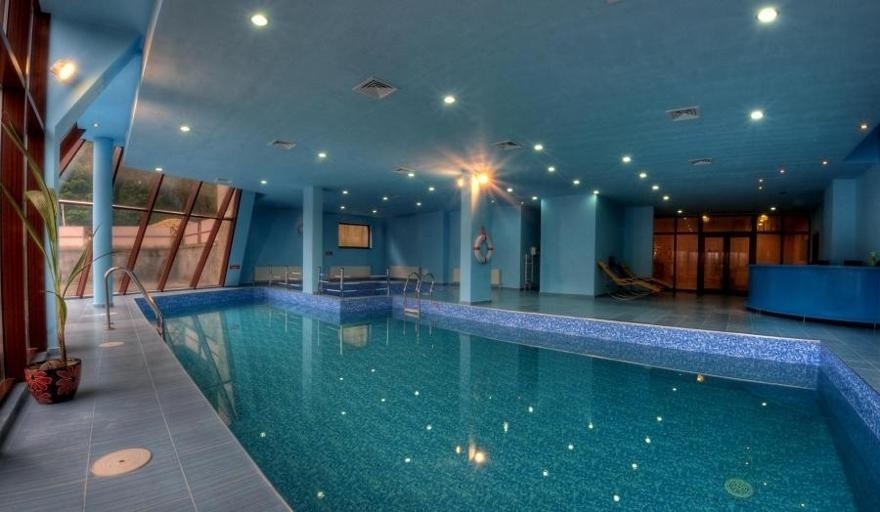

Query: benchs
[449,267,502,290]
[327,265,371,282]
[253,264,303,286]
[379,265,422,280]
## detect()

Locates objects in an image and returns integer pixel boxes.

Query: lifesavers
[474,233,492,262]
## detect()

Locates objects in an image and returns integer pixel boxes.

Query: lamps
[49,57,77,84]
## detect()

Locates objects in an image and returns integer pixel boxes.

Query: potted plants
[0,110,129,406]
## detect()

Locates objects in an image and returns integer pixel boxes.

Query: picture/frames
[335,221,370,249]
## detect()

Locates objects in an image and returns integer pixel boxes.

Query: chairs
[598,259,673,304]
